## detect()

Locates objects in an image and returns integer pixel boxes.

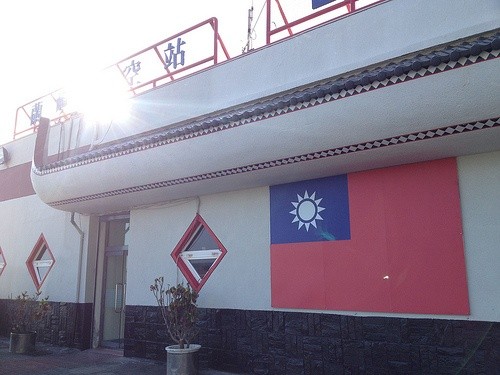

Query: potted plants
[5,290,50,354]
[148,276,202,375]
